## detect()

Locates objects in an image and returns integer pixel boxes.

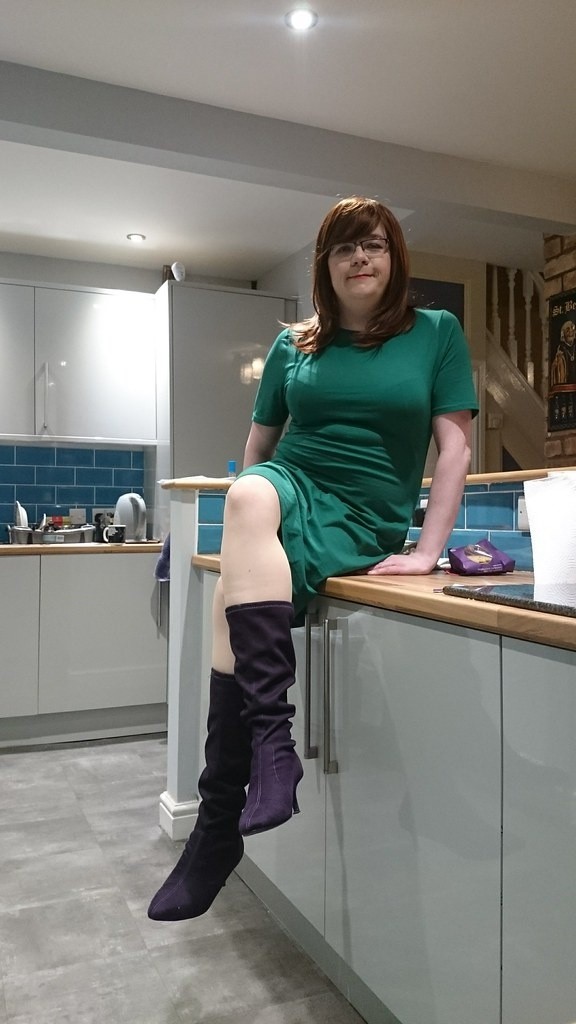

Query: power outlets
[92,508,116,525]
[69,508,87,524]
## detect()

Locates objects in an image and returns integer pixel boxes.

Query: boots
[225,600,304,837]
[148,667,250,922]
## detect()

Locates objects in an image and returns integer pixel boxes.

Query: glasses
[329,238,390,258]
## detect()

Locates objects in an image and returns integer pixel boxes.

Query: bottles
[568,393,573,417]
[561,395,567,418]
[553,396,559,421]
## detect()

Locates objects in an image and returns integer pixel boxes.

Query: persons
[148,196,480,920]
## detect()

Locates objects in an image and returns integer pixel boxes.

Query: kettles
[113,493,147,541]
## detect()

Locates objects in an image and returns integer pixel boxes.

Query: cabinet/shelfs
[191,556,576,1024]
[0,280,296,719]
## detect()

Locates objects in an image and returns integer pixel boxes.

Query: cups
[103,525,126,543]
